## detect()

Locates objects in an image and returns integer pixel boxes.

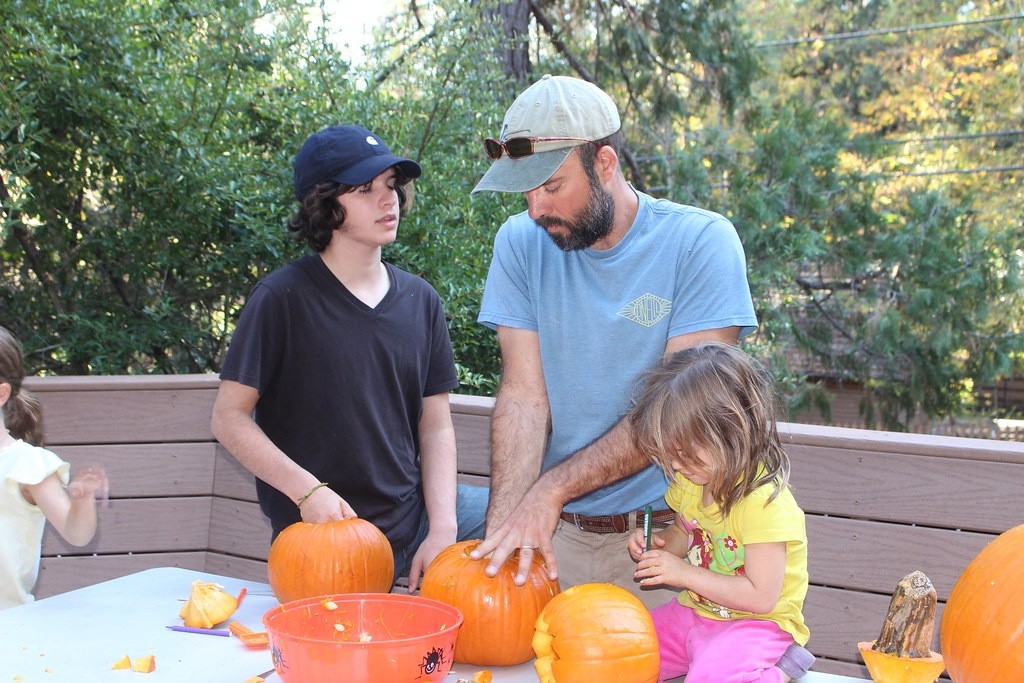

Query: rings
[522,546,532,549]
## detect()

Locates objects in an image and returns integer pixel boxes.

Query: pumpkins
[941,524,1024,683]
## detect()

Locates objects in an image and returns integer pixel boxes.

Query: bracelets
[297,482,329,507]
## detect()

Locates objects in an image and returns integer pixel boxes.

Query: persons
[211,124,490,594]
[0,326,108,612]
[627,344,816,683]
[469,73,759,611]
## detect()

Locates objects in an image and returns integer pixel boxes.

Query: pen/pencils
[643,504,653,554]
[166,626,232,637]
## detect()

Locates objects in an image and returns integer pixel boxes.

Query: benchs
[17,374,1024,682]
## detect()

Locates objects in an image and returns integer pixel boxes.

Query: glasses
[484,137,600,161]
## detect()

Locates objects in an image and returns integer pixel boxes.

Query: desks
[1,567,875,683]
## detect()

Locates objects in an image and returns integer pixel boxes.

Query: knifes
[243,668,275,683]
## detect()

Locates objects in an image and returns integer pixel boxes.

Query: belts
[561,508,678,534]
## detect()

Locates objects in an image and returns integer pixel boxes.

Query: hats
[295,126,422,202]
[471,74,621,196]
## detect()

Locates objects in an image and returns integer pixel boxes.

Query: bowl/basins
[260,592,463,683]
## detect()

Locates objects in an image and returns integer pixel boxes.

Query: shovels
[228,622,270,647]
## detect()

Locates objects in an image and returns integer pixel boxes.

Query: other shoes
[777,645,816,680]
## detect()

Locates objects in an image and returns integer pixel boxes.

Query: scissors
[245,669,276,683]
